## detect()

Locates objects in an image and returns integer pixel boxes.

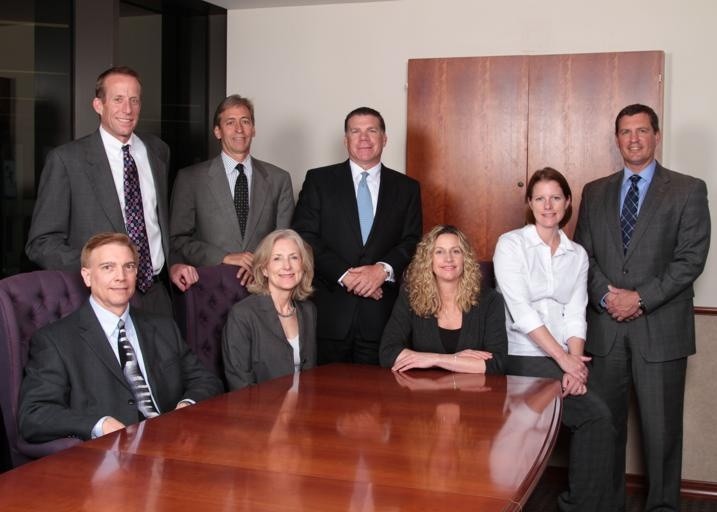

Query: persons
[24,67,199,320]
[293,107,423,366]
[168,96,296,289]
[18,232,225,445]
[492,167,620,507]
[573,103,711,508]
[221,228,318,389]
[379,224,509,374]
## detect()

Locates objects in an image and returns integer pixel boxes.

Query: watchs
[638,292,647,307]
[380,262,393,274]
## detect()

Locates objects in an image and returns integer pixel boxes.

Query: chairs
[478,260,495,286]
[180,260,251,385]
[0,269,92,465]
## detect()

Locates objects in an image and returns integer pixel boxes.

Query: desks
[0,361,568,511]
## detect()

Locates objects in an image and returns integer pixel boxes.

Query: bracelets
[451,354,460,373]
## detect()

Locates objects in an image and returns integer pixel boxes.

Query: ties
[619,173,641,253]
[233,163,249,240]
[120,143,154,297]
[356,171,374,248]
[115,318,161,419]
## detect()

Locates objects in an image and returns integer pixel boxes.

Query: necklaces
[273,306,295,316]
